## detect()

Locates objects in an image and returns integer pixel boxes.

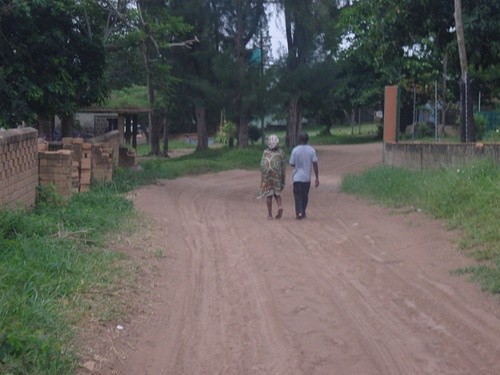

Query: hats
[268,135,279,149]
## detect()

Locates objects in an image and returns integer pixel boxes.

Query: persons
[289,133,319,219]
[257,135,286,220]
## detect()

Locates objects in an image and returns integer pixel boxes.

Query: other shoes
[275,208,283,219]
[267,217,272,220]
[296,213,302,218]
